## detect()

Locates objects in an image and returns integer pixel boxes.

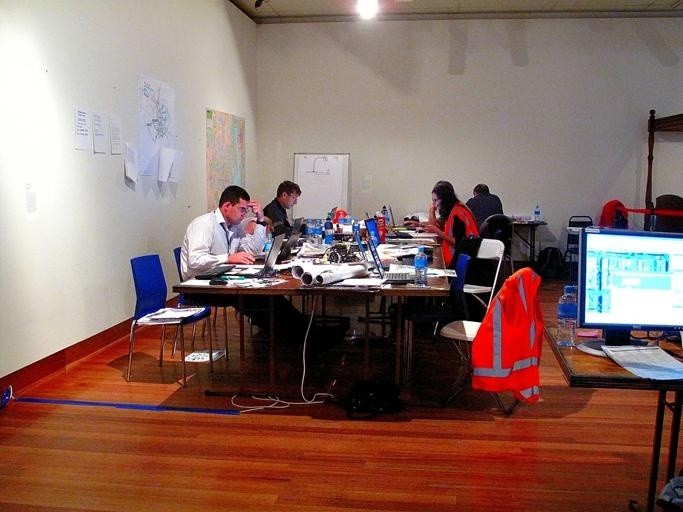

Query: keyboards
[394,232,413,238]
[195,266,236,279]
[403,257,415,266]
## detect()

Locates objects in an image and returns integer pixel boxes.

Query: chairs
[433,238,505,337]
[477,214,514,279]
[650,194,683,234]
[563,216,594,283]
[171,247,245,361]
[439,267,541,415]
[126,254,215,387]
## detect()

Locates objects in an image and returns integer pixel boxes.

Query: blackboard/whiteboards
[292,153,350,219]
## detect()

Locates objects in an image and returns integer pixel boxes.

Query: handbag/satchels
[342,380,402,416]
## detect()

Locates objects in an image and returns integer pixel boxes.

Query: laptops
[280,217,304,250]
[364,210,397,238]
[388,204,401,227]
[366,236,415,284]
[254,231,302,262]
[235,233,285,278]
[322,207,337,240]
[364,218,381,249]
[353,230,401,270]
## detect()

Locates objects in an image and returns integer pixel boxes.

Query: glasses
[433,198,440,202]
[289,194,299,200]
[237,207,250,213]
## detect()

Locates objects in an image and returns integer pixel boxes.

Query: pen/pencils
[425,243,440,246]
[220,276,244,281]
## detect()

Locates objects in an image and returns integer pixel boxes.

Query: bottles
[324,217,333,244]
[338,212,344,222]
[306,216,313,242]
[414,246,428,285]
[352,218,360,241]
[385,212,390,224]
[314,220,323,245]
[534,205,541,223]
[556,286,578,346]
[265,233,272,263]
[344,211,351,224]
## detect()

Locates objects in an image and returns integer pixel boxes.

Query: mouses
[209,278,228,285]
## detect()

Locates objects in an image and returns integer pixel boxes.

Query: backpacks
[537,247,565,280]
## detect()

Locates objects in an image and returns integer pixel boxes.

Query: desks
[173,221,452,403]
[512,220,548,272]
[543,320,683,511]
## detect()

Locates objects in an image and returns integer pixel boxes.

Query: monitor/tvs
[576,228,683,358]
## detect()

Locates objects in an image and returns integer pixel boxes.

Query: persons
[180,183,333,385]
[464,183,504,239]
[406,180,480,337]
[241,215,275,236]
[258,180,302,229]
